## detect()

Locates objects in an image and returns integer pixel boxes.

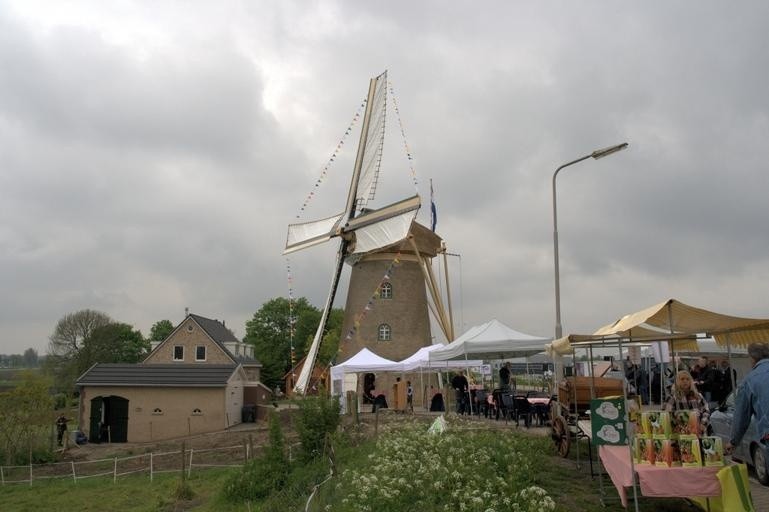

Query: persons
[451,369,468,416]
[55,412,74,447]
[498,361,511,389]
[662,369,712,446]
[406,380,414,412]
[722,341,769,482]
[365,388,389,412]
[625,353,737,411]
[75,429,87,445]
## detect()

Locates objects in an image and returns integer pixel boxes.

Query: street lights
[550,142,630,393]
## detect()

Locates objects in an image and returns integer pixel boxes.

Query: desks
[578,420,601,481]
[594,441,728,510]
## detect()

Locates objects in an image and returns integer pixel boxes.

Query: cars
[711,381,768,487]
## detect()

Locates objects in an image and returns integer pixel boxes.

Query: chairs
[456,383,556,427]
